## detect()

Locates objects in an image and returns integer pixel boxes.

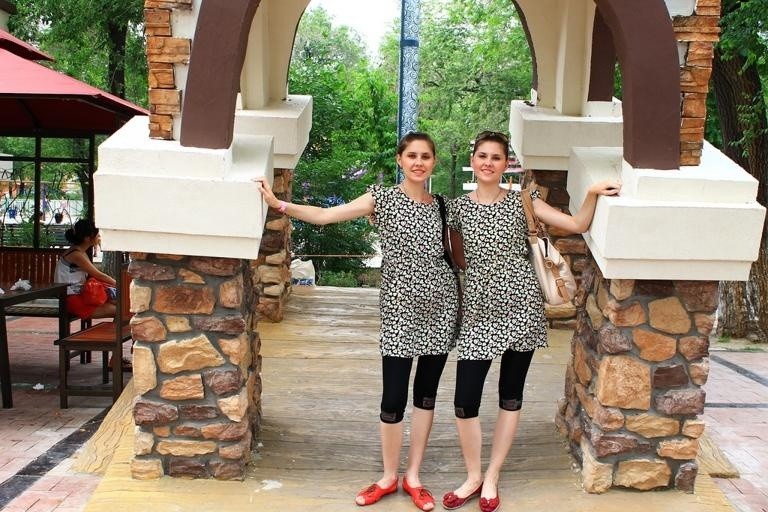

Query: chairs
[54,260,136,409]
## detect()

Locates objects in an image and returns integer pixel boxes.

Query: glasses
[475,130,509,144]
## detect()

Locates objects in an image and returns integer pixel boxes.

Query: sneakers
[402,475,435,511]
[480,480,500,512]
[356,476,399,506]
[442,480,482,510]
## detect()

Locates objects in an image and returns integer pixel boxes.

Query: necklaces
[399,179,427,205]
[473,189,504,205]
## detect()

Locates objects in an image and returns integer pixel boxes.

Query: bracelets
[275,198,289,215]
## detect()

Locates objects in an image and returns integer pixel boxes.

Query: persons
[49,212,69,226]
[29,210,47,225]
[440,127,622,512]
[55,216,134,372]
[251,131,461,512]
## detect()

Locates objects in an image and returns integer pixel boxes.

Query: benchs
[0,247,92,371]
[0,224,74,249]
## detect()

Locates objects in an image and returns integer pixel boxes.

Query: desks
[0,281,73,408]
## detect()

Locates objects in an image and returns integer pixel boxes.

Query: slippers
[108,359,132,373]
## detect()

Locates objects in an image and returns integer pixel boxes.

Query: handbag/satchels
[82,276,108,306]
[527,235,577,307]
